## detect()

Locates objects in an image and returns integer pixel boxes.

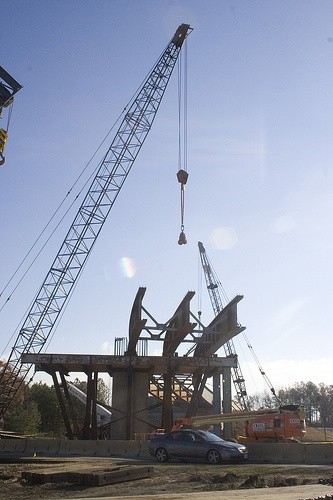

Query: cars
[147,427,249,464]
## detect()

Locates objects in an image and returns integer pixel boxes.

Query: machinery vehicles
[194,240,308,444]
[59,376,112,439]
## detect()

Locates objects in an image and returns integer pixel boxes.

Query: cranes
[0,22,194,421]
